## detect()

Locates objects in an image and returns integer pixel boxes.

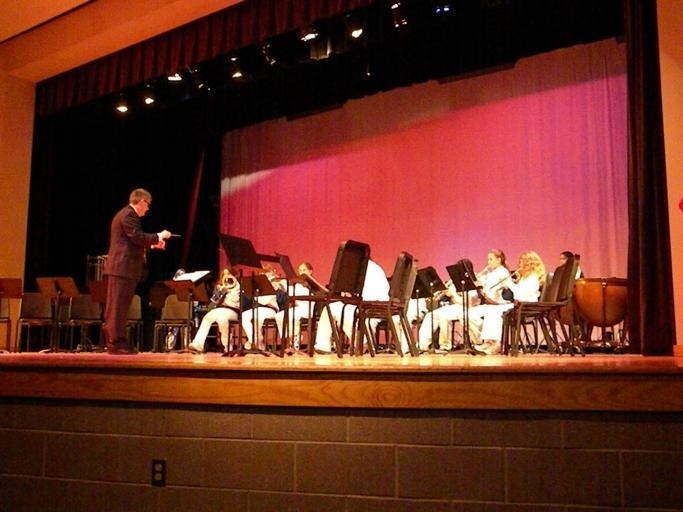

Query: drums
[572,279,631,352]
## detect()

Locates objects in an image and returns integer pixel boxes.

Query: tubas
[212,275,237,305]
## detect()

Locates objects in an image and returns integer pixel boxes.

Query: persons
[458,249,512,344]
[184,267,241,355]
[274,262,316,352]
[549,251,581,280]
[419,259,477,354]
[314,259,391,354]
[471,251,544,354]
[391,297,426,354]
[134,240,169,351]
[239,264,286,350]
[102,187,171,353]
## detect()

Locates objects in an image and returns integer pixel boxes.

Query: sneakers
[472,340,501,355]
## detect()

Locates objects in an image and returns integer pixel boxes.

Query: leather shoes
[109,345,138,355]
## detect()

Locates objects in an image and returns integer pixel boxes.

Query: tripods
[222,270,251,358]
[426,283,449,355]
[244,289,270,359]
[405,290,426,356]
[452,284,475,357]
[461,289,487,356]
